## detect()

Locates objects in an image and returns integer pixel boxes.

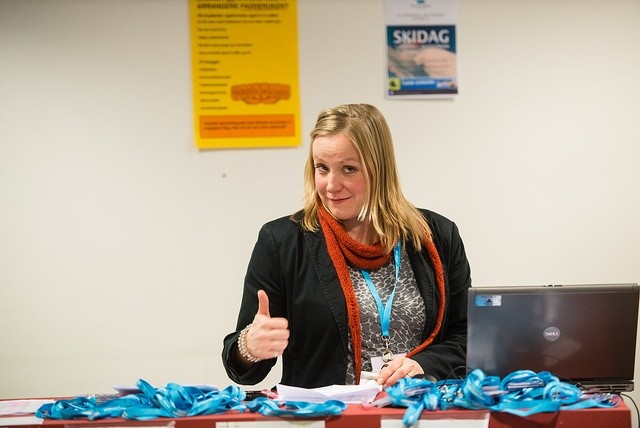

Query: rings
[408,372,414,377]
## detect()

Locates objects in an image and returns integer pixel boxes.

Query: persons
[223,103,472,407]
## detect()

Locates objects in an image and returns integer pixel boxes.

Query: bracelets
[238,324,260,362]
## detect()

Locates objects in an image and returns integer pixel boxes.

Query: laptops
[466,283,640,379]
